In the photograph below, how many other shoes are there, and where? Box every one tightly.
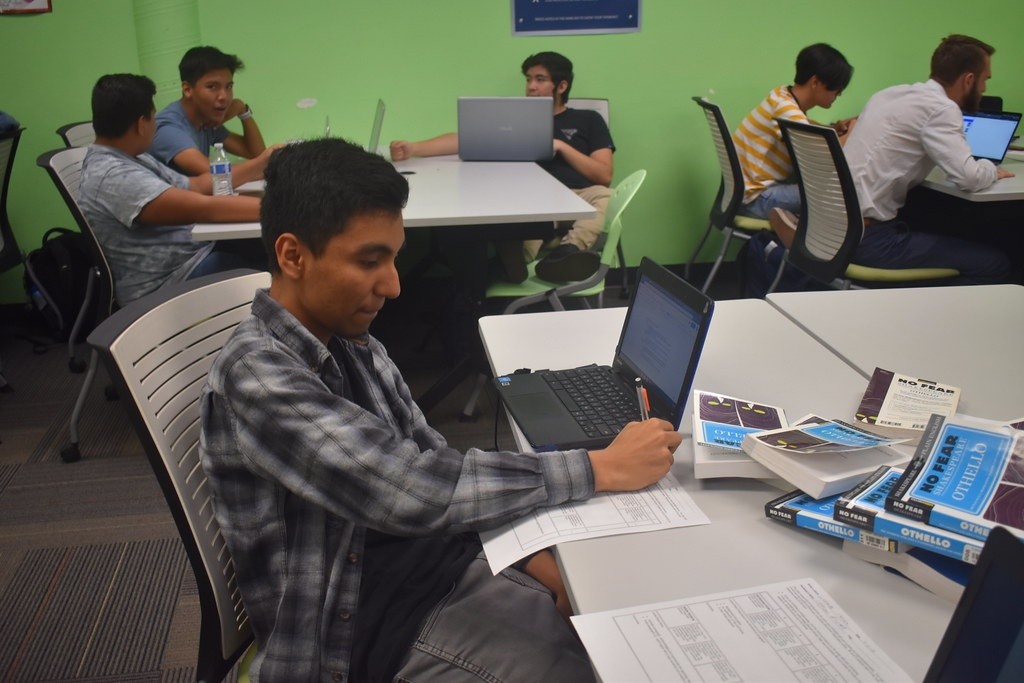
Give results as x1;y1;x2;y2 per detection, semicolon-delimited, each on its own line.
536;243;601;283
495;239;528;283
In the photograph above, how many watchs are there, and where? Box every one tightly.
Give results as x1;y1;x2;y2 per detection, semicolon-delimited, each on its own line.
238;105;253;120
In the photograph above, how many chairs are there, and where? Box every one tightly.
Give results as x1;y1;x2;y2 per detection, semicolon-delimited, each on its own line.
37;143;112;461
0;111;31;398
53;117;100;373
459;166;651;423
561;95;629;298
767;119;962;298
86;268;272;681
683;94;787;295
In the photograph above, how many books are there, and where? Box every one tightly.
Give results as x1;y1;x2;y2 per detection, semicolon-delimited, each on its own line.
691;365;1024;603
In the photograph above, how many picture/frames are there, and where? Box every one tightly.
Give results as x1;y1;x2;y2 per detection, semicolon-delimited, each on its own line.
0;0;52;15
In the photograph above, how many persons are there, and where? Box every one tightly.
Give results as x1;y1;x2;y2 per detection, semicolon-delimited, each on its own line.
389;51;616;283
76;73;287;308
145;45;267;177
196;139;684;683
824;34;1013;284
728;42;859;220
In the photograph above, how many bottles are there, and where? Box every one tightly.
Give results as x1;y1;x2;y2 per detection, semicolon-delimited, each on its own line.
209;142;233;196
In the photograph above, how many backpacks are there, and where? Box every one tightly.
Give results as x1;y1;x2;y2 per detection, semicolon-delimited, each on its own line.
23;228;100;342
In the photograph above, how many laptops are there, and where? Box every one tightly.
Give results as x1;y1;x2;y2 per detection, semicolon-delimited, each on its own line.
496;255;717;450
963;107;1024;164
457;97;557;161
368;99;385;154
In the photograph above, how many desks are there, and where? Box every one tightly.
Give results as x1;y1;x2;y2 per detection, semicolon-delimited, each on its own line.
475;297;973;682
765;281;1023;478
192;159;597;377
925;145;1024;202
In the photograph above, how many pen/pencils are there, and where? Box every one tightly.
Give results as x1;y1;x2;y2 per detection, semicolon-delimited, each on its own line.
635;377;650;421
